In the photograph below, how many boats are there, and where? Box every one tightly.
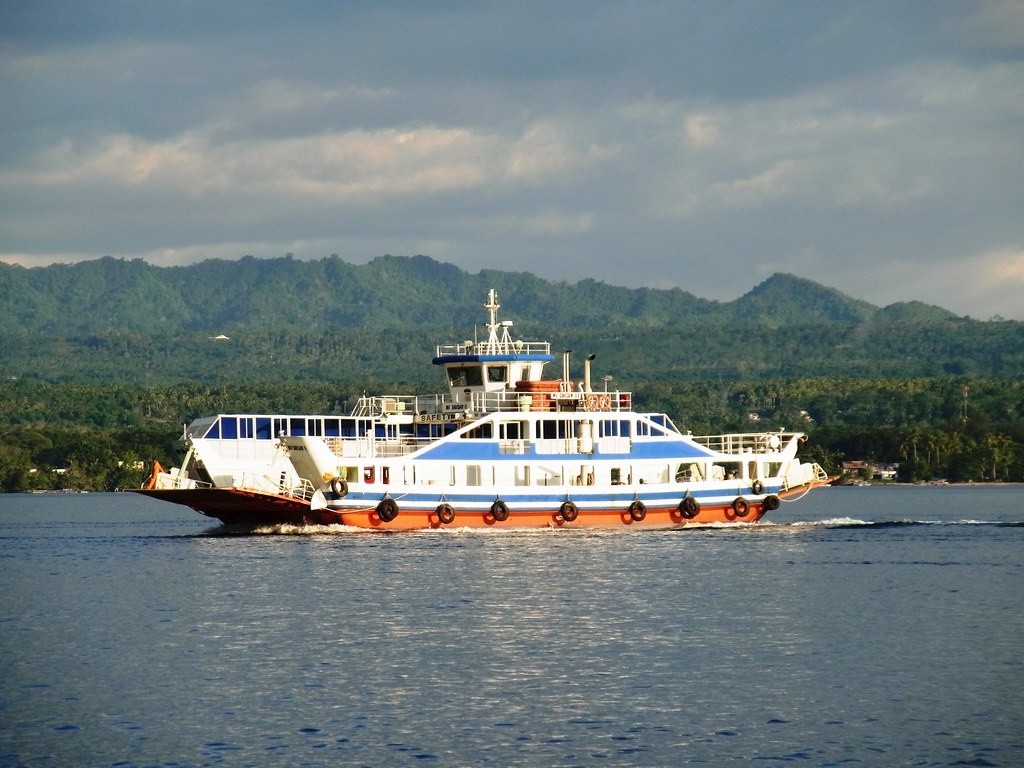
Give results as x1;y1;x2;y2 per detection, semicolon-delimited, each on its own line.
118;288;842;531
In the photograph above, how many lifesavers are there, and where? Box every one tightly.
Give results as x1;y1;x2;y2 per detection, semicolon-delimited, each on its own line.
797;435;810;452
492;501;509;521
585;393;611;412
765;495;780;510
752;481;764;495
330;476;348;497
733;497;750;517
561;502;579;522
377;499;399;522
680;497;700;519
630;501;646;521
438;504;455;524
